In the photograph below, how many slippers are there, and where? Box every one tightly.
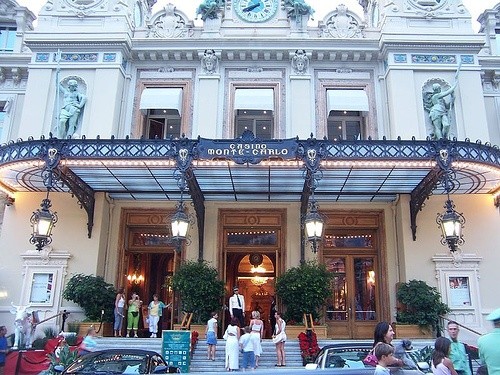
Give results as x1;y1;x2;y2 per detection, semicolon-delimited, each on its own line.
281;364;286;366
275;364;281;367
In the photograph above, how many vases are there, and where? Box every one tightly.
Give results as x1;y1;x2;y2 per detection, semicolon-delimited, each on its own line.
304;353;316;366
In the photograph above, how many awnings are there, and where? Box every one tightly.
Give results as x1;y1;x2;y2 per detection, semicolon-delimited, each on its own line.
140;88;183;118
233;88;274;116
326;89;369;120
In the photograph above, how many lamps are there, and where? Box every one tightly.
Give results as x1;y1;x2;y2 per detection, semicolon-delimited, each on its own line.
435;159;466;251
28;148;97;252
167;154;203;264
126;274;144;289
298;152;327;264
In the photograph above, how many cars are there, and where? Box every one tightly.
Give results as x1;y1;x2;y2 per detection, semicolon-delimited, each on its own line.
60;349;180;375
305;343;430;375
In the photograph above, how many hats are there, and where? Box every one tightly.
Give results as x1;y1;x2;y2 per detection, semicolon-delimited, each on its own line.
486;308;500;321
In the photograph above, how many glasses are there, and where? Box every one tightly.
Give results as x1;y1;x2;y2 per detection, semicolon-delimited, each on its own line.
235;290;238;291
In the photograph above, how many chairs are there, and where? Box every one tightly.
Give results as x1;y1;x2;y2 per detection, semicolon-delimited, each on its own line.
330;356;343;366
100;358;123;372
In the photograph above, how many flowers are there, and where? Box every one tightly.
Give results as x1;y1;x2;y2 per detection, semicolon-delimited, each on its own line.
297;330;321;356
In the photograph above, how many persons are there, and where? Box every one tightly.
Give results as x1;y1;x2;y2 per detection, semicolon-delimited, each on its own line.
125;292;143;338
272;311;287;367
57;66;87;138
204;55;217;72
146;294;172;337
79;328;98;355
430;73;459;139
238;310;264;374
432;321;472;375
205;312;218;361
294;54;306;73
224;316;240;371
113;287;127;337
477;309;500;375
373;322;403;375
229;286;246;335
0;326;9;375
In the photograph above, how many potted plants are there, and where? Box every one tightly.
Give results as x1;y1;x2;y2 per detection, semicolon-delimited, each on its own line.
390;278;447;340
60;272;119;336
167;261;227;341
274;263;336;340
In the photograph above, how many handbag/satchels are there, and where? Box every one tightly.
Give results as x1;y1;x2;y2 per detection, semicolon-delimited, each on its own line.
241;349;243;353
131;312;138;317
363;342;382;367
223;329;228;340
273;332;285;343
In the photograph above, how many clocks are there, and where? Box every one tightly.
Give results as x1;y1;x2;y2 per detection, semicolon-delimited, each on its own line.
231;0;279;23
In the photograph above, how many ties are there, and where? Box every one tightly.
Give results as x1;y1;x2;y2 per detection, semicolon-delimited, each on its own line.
237;295;241;307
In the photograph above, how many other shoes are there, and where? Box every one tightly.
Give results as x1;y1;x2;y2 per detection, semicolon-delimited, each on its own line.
134;334;138;337
154;334;157;338
127;334;129;337
150;333;154;337
